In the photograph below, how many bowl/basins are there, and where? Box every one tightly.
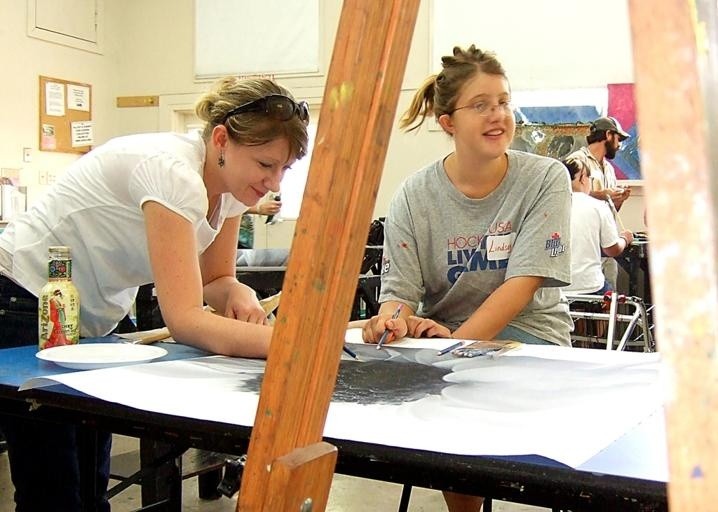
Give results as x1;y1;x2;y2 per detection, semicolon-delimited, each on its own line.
235;248;289;267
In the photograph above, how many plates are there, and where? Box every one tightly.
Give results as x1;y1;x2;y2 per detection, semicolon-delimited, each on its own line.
33;341;170;373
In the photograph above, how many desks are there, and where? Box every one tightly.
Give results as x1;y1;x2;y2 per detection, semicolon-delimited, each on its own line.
0;326;670;512
600;234;650;345
234;265;381;323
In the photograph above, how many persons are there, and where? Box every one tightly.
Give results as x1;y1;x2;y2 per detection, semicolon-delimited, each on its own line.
361;44;579;511
242;195;284;219
0;74;317;511
568;114;633;217
559;156;635;318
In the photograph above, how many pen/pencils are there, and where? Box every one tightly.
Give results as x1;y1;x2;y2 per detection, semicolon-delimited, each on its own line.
343;344;359;360
450;342;503;358
376;303;404;350
436;341;465;356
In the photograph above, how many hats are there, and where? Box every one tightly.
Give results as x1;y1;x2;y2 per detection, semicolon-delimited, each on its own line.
590;118;631;142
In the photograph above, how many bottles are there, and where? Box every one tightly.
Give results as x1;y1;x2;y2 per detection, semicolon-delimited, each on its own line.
36;244;81;347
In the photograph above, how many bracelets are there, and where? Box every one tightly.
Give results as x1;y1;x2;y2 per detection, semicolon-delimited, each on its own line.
619;235;630;247
257;203;261;214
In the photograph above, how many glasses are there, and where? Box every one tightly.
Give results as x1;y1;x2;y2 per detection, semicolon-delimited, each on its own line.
221;94;309;127
452;101;515;116
